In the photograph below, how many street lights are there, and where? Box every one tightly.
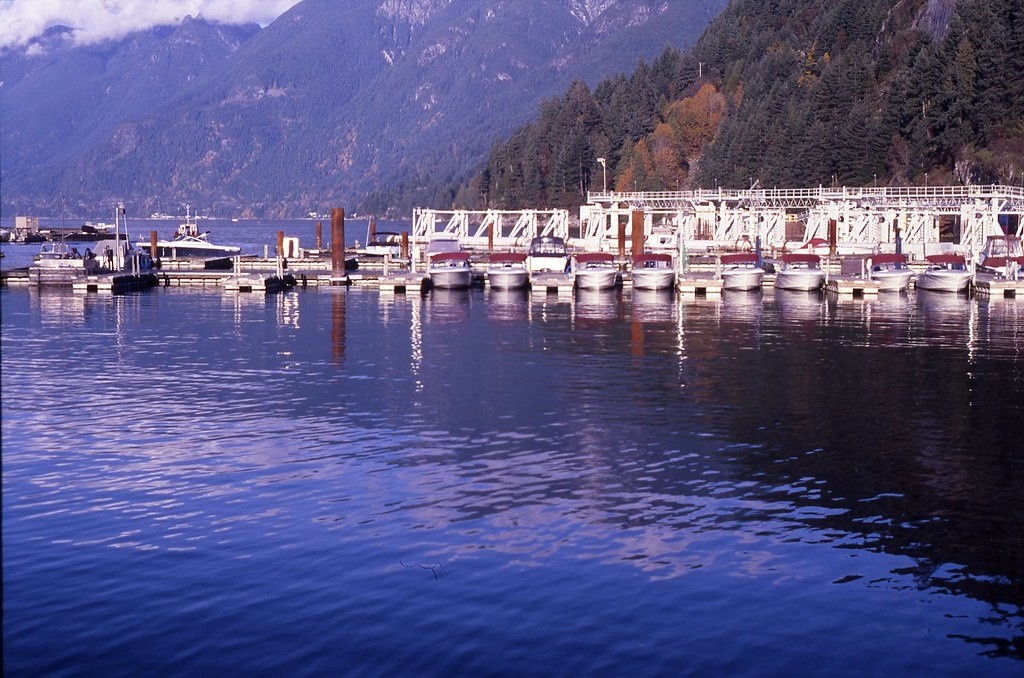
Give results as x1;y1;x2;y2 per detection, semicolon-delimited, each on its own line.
597;158;606;197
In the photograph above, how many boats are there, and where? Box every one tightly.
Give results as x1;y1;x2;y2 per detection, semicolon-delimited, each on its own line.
572;253;618;291
865;254;915;293
486;252;527;291
720;253;765;291
526;236;567;272
774;254;824;292
429;251;471;291
33;243;96;269
630;253;676;291
915;254;972;293
978;235;1024;279
136;203;241;270
365;216;403;257
